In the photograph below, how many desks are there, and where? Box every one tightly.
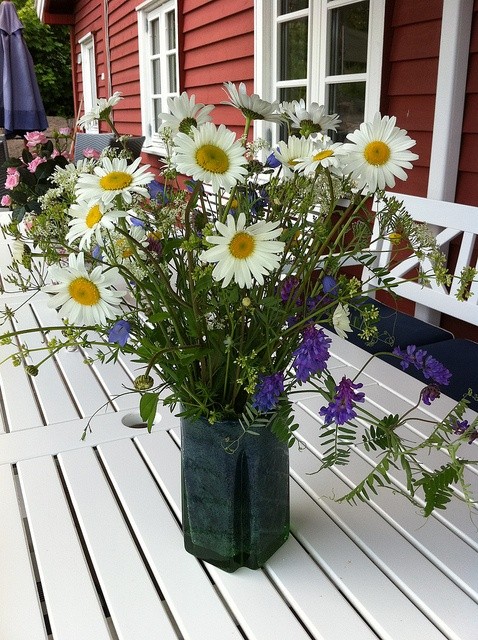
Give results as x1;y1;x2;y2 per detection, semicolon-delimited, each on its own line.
0;208;477;640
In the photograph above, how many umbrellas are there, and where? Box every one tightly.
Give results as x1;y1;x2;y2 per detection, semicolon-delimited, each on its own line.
0;2;49;138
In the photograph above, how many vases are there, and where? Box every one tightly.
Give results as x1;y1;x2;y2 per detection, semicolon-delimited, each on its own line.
14;214;47;246
181;407;291;571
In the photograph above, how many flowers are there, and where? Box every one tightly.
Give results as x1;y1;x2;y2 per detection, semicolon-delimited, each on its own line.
0;82;478;501
0;125;100;216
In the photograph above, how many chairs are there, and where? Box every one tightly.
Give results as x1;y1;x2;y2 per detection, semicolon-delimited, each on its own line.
111;136;142;168
74;132;113;168
338;295;456;363
392;338;477;413
